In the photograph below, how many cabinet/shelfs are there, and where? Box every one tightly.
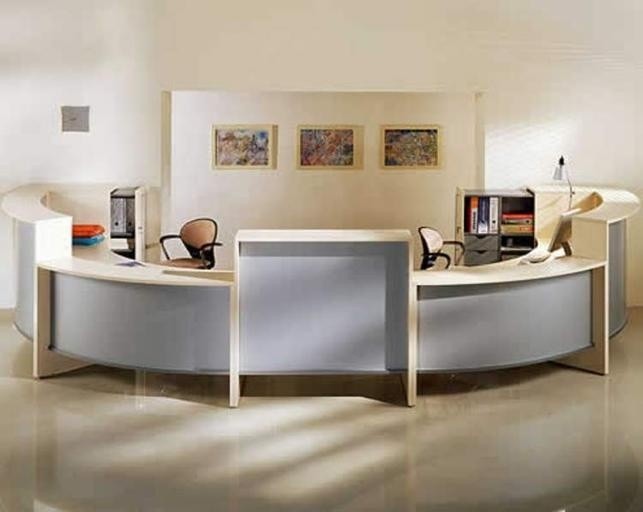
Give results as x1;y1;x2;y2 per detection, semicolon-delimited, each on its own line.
110;188;143;261
456;189;536;266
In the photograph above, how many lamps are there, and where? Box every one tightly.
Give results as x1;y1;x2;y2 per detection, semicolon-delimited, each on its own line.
554;157;577;211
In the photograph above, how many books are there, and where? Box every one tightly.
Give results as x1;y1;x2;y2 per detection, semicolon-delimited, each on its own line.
500;210;533;235
487;197;499;235
476;197;488;235
466;196;479;234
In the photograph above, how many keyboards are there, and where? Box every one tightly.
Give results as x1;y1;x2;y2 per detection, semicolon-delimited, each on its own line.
527;254;550;263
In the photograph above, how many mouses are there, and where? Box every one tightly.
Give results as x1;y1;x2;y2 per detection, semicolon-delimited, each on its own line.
520;259;529;265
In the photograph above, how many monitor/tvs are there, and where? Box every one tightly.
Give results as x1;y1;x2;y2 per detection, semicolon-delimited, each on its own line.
547;208;582;260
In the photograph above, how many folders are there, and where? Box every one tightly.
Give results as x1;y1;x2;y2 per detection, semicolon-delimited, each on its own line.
468;197;501;234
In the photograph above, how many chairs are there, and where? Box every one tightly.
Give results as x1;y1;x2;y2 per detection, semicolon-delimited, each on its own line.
417;227;466;271
161;218;223;269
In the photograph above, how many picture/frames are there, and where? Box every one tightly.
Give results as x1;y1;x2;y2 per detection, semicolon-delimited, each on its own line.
296;123;365;170
379;124;444;171
211;124;277;167
